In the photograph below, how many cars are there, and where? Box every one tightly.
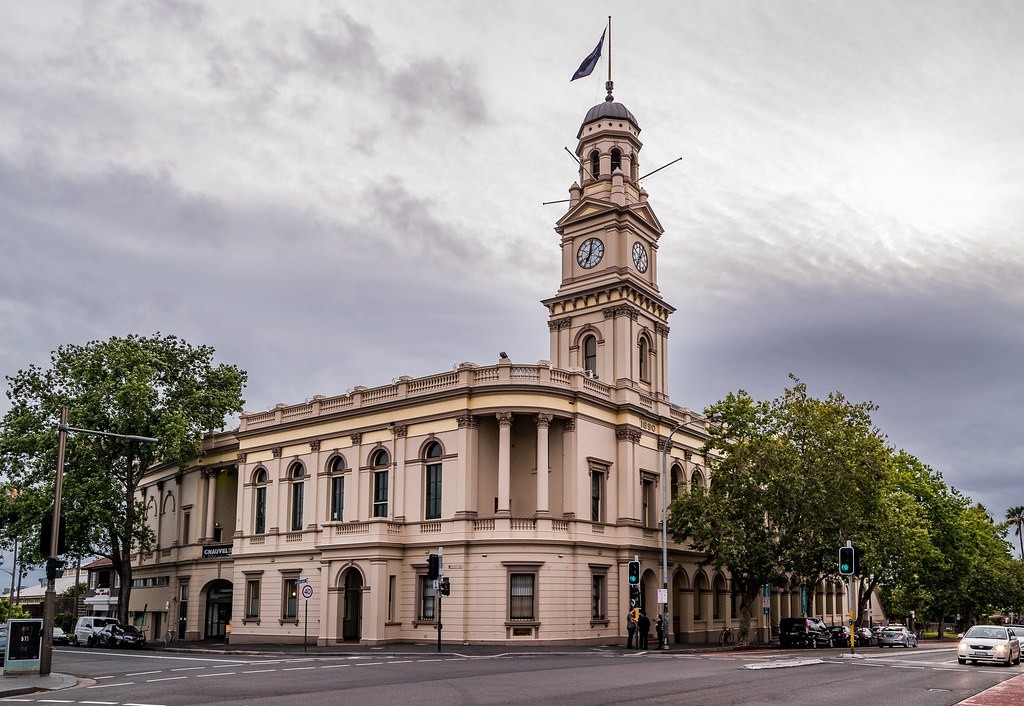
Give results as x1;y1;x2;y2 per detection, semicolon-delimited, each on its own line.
53;627;70;646
826;625;918;648
0;624;8;666
957;624;1024;667
94;624;147;649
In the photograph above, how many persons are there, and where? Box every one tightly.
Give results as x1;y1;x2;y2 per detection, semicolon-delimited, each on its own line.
654;614;663;649
627;608;636;649
637;612;650;649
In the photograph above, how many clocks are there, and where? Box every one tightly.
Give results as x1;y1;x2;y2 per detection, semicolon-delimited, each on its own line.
577;238;605;269
632;241;648;274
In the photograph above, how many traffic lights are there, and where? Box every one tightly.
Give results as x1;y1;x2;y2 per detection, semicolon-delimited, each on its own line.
630;587;638;599
839;547;854;576
426;553;439;580
441;581;451;595
629;561;640;585
55;561;66;578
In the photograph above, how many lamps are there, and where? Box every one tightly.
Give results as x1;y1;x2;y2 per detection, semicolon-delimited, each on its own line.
583;370;593;378
499;351;508;359
593;374;598;378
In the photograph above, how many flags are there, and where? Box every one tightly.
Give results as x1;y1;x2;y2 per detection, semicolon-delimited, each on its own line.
570;28;607;82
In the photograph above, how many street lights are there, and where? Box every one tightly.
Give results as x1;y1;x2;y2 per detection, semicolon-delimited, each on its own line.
662;413;722;650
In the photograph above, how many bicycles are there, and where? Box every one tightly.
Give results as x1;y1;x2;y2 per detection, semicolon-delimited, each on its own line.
719;627;734;647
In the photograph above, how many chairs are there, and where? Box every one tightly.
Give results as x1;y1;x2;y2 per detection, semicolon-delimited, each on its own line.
996;631;1005;637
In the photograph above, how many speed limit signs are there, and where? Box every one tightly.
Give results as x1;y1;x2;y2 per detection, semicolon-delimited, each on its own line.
301;585;315;599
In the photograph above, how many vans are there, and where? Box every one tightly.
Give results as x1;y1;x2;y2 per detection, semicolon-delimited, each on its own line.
779;617;834;649
73;617;122;647
